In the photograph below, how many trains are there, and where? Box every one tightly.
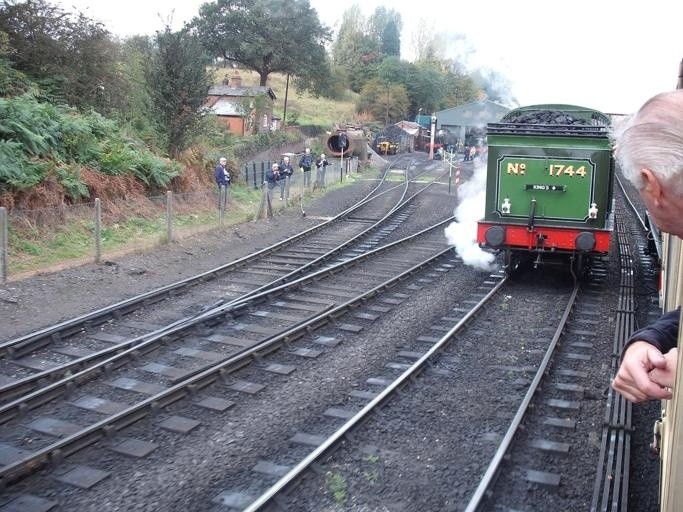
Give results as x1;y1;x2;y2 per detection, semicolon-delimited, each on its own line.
476;103;617;277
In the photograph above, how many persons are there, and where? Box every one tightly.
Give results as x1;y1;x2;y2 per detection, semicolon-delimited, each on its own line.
215;157;232;209
298;147;314;187
445;140;483;162
264;163;282;203
606;88;683;405
315;153;334;188
278;157;294;201
